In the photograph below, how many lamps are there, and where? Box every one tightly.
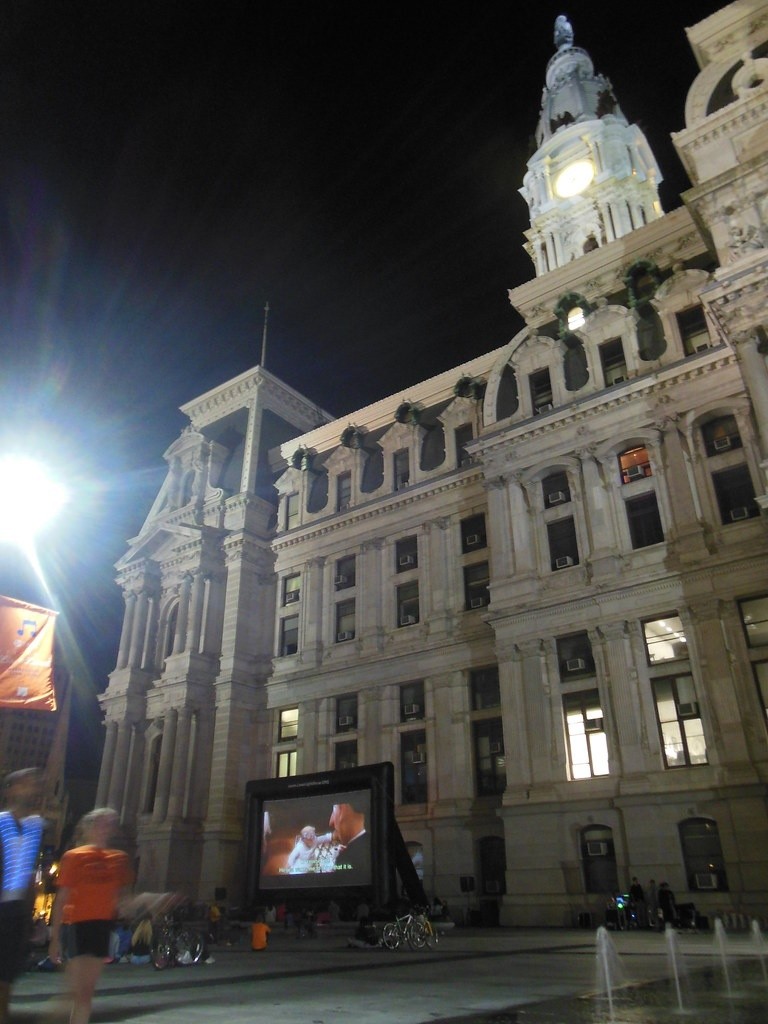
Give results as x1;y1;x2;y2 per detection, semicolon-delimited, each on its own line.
341;426;356;447
454;377;475;397
288;448;305;470
394;402;412;423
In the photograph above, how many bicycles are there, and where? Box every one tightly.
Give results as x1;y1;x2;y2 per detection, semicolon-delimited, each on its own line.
149;914;208;969
382;902;442;953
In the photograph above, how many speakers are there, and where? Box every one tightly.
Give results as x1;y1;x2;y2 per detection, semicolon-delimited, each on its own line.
460;877;474;892
215;887;227;900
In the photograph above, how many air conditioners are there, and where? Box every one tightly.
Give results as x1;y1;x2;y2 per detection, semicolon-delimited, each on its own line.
678;703;697;716
549;491;565;503
695;343;709;353
539;404;552;414
467;534;479;545
491;742;500;753
338;632;351;641
339;717;353;725
400;555;413;565
412;752;425;763
696;874;717;888
405;704;419;714
556;556;574;568
401;615;415;625
398;482;407;490
587;843;607;855
585;718;603;732
627;465;644;477
714;436;731;450
334;575;346;584
567;659;585;671
731;507;749;520
286;592;297;602
613;375;625;385
486;880;503;893
461;458;472;466
471;598;485;608
340;504;349;512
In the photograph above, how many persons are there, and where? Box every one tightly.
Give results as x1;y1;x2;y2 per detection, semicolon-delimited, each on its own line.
2;769;43;1023
284;803;365;876
50;808;134;1022
607;876;679;932
